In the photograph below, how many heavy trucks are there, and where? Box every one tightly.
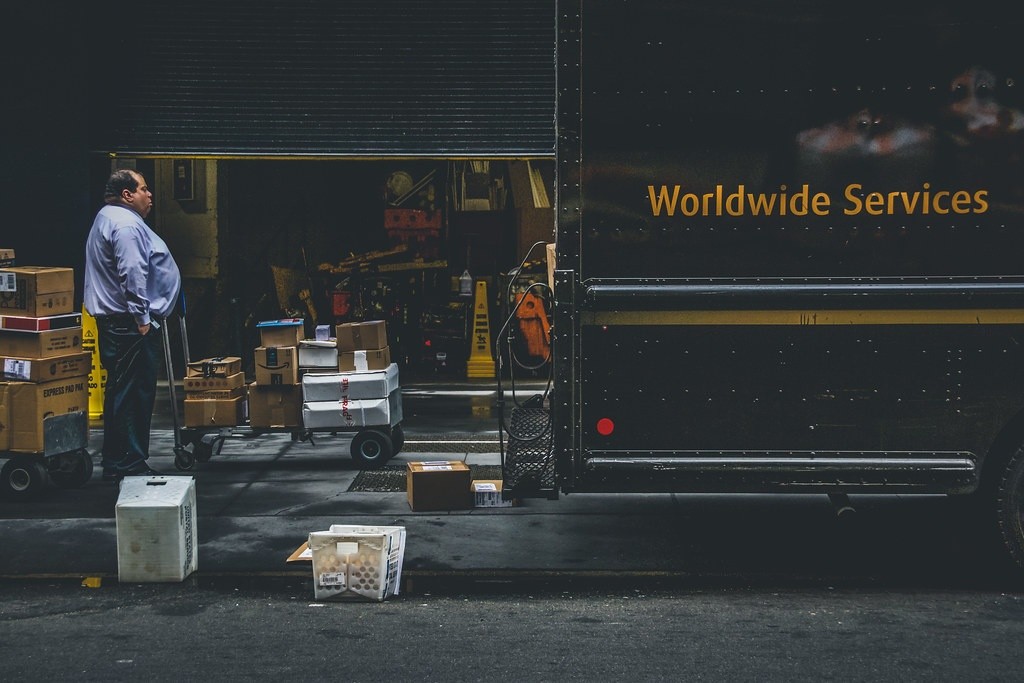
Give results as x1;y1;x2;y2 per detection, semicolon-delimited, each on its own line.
0;0;1024;590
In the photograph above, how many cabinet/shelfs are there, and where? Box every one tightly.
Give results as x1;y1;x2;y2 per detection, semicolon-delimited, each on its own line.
457;207;554;276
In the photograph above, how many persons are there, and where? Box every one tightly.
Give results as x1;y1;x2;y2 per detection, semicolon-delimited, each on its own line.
83;168;181;478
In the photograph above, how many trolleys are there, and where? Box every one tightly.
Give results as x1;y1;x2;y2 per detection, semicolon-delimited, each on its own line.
160;288;405;471
0;256;93;500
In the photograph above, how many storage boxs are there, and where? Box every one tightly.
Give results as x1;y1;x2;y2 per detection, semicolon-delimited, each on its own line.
470;477;503;507
405;459;472;513
331;290;352;317
183;320;404;429
381;207;441;244
115;476;197;583
308;524;407;602
0;249;93;454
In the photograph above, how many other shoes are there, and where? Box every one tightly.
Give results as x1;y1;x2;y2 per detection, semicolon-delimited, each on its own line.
103;468;161;481
